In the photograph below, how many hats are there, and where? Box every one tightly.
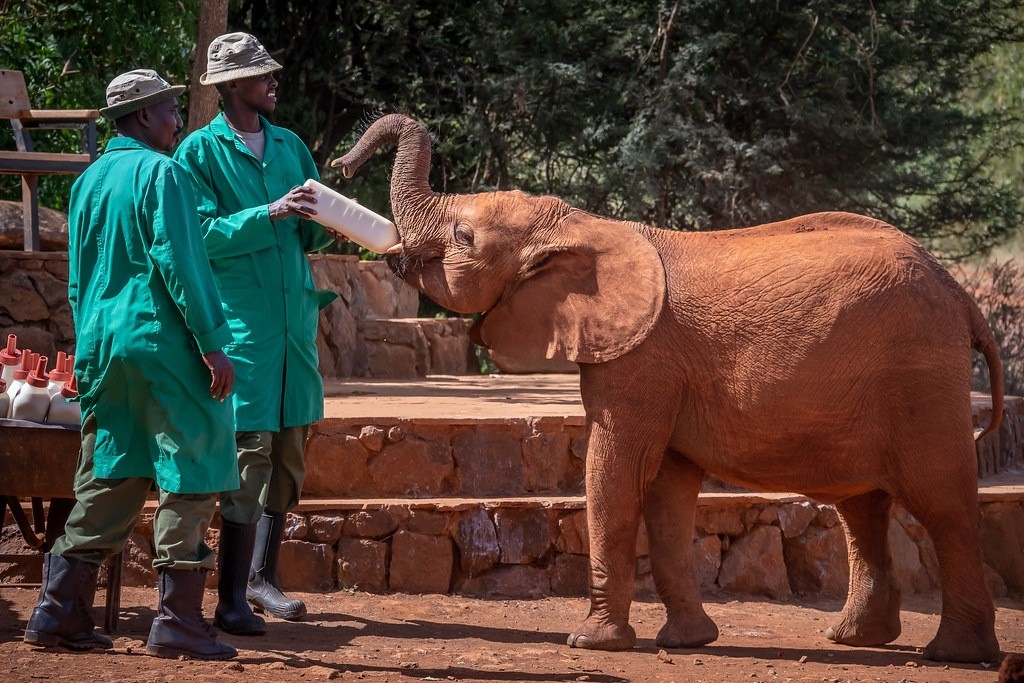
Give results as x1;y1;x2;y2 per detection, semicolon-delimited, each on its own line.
200;32;283;85
99;69;187;121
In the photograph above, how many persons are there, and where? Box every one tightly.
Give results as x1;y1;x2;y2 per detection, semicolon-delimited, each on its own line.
25;69;238;660
170;32;358;636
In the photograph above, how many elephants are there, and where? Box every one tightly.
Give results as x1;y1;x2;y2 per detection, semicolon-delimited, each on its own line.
330;113;1006;663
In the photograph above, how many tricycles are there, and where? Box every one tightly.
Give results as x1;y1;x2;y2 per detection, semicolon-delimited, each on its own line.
0;418;124;635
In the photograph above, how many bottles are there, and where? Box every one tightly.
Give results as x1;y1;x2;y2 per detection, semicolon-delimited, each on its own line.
295;179;397;255
0;334;82;425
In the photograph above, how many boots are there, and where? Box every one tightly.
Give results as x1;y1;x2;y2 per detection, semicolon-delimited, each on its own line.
245;513;307;621
145;567;238;661
213;515;267;636
24;554;114;649
